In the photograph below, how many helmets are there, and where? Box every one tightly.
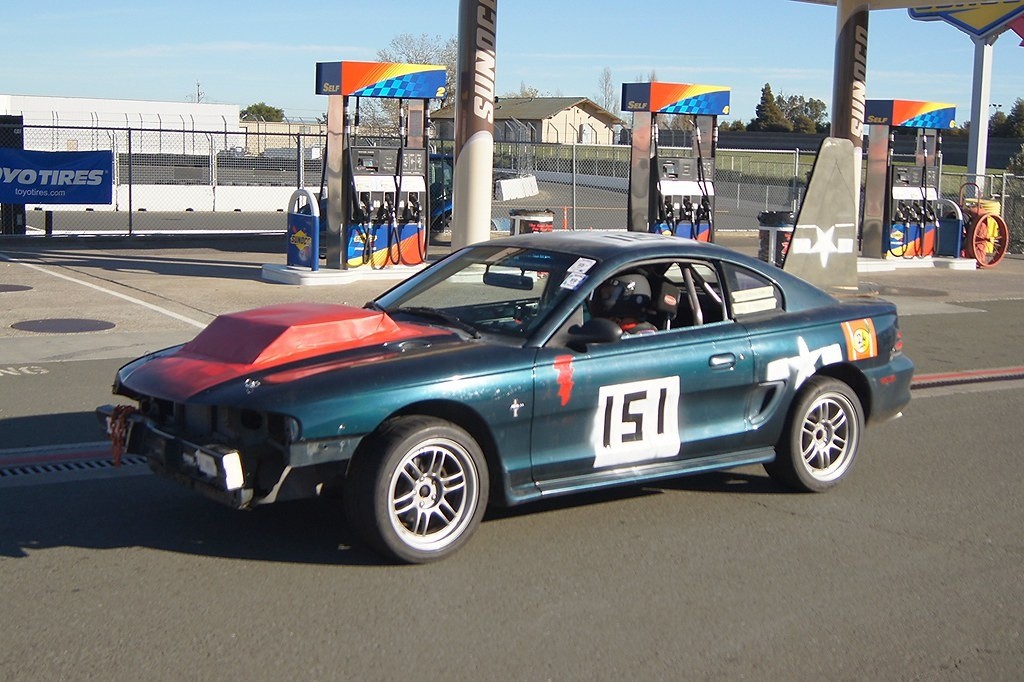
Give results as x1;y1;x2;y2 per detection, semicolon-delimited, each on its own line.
597;273;651;323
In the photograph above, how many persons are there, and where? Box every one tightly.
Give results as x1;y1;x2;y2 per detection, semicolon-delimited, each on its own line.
513;269;660;350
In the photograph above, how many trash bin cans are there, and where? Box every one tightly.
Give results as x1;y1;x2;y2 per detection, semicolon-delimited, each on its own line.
759;212;793;267
509;210;553;236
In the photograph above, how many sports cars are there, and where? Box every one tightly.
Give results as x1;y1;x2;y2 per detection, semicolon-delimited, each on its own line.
94;231;916;565
